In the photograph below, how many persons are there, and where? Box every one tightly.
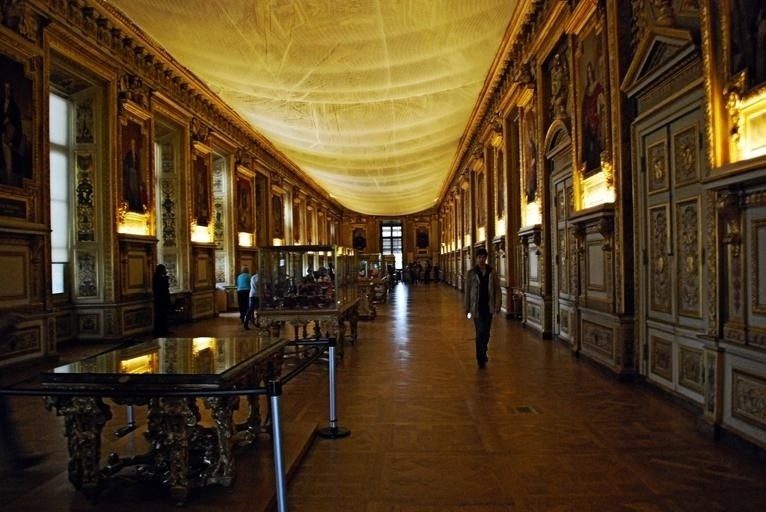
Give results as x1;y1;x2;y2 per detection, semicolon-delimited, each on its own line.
0;75;23;190
353;231;365;251
416;228;428;248
580;60;602;173
243;268;265;331
0;307;48;481
463;247;503;369
123;137;144;210
150;263;176;331
236;266;250;324
196;169;207;215
237;183;251;227
305;257;440;294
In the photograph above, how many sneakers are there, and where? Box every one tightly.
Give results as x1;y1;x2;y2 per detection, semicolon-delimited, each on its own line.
477;356;488;368
239;315;252;331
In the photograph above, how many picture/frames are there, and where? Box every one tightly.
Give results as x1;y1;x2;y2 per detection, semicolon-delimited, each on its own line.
351;226;368;253
490;133;506;236
0;25;50;236
235;164;256;247
116;98;155;235
271;183;285;247
414;222;431;249
189;140;214;244
516;83;543;227
560;0;618;210
698;0;766;184
